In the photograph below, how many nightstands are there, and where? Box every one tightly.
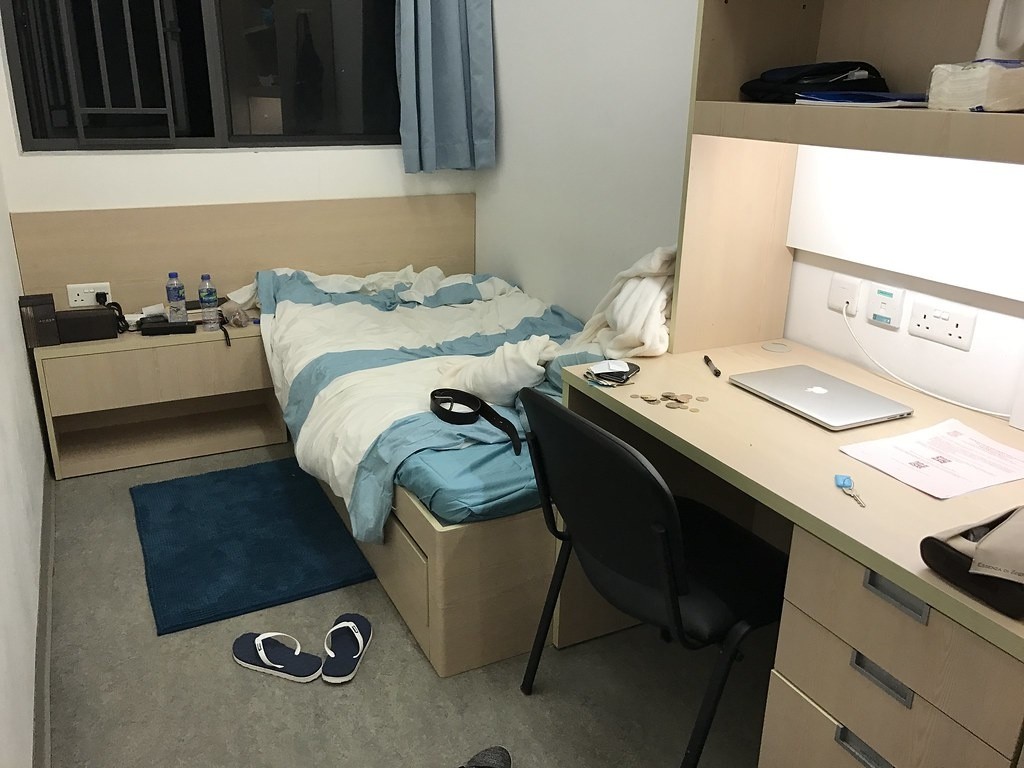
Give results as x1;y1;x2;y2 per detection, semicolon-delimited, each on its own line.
33;307;287;481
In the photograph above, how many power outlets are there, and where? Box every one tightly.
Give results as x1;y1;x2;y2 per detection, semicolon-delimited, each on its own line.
909;300;976;352
67;282;112;307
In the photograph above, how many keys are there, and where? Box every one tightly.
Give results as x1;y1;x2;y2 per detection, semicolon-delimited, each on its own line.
841;487;866;508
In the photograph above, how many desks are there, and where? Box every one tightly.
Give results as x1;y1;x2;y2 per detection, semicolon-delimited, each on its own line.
553;337;1024;663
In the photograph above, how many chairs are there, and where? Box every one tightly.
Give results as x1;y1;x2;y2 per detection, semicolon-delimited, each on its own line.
520;383;788;768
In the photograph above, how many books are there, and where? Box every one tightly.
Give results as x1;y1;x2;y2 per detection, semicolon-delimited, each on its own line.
794;91;929;108
596;362;640;383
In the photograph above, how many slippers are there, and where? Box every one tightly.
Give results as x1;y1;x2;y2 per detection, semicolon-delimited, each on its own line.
321;613;373;684
231;632;324;684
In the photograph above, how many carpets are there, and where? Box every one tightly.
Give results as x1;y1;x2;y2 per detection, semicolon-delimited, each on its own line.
129;458;377;636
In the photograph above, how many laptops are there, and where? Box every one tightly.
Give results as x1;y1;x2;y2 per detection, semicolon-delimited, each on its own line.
728;364;915;432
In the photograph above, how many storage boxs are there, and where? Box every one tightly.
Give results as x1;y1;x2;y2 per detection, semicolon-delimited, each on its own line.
55;307;118;343
18;293;60;349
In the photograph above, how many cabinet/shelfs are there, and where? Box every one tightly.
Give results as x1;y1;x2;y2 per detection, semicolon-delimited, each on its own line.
552;0;1024;768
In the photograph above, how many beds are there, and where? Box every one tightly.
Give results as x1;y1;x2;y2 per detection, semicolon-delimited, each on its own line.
255;264;607;679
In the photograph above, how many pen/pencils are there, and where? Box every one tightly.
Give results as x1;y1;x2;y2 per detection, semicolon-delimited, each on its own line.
704;355;721;377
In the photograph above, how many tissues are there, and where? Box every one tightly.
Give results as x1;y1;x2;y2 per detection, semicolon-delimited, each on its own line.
926;57;1024;113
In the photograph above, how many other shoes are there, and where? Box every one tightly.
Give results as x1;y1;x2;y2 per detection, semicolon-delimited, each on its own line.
456;746;512;768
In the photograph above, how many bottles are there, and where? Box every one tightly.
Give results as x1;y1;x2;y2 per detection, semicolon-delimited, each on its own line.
198;274;220;332
165;272;188;322
